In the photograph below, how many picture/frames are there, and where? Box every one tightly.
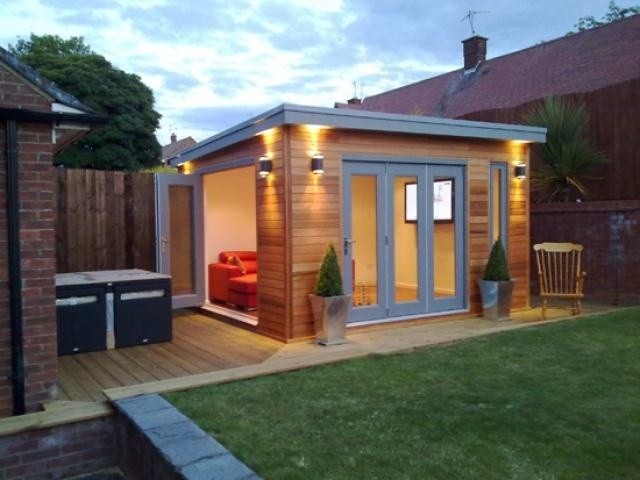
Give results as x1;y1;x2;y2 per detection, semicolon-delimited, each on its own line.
404;178;455;223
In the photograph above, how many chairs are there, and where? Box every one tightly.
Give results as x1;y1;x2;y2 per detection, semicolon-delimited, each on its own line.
533;242;587;321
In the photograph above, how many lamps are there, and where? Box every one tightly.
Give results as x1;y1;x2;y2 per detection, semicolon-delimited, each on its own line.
177;164;185;175
311;156;325;174
256;157;273;177
515;164;527;180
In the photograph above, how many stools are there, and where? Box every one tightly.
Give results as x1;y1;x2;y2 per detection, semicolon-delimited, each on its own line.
228;273;259;310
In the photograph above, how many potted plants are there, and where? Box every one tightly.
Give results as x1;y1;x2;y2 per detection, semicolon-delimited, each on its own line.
477;236;516;322
307;240;353;345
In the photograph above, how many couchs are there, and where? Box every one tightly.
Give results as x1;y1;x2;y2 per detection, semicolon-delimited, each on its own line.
208;251;259;308
